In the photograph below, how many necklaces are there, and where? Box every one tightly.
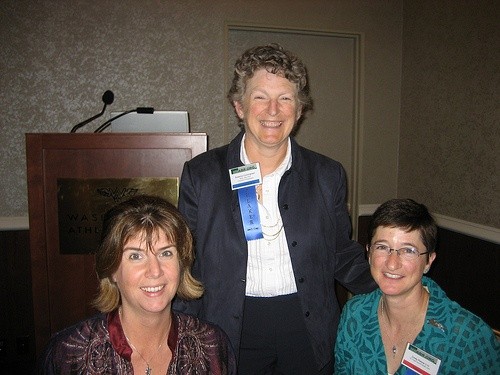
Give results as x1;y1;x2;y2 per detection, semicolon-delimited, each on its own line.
256;189;263;202
381;297;428;357
258;217;284;240
117;310;172;375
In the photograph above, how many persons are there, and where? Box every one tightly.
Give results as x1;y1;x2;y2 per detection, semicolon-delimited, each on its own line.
38;194;237;375
333;198;500;375
171;41;381;375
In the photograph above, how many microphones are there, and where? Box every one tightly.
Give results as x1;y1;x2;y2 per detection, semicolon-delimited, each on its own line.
71;90;114;132
93;107;155;133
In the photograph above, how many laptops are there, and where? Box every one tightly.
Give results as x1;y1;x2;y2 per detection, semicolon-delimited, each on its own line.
110;110;189;133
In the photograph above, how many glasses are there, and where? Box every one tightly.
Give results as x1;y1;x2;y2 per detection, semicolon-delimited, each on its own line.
368;243;430;261
